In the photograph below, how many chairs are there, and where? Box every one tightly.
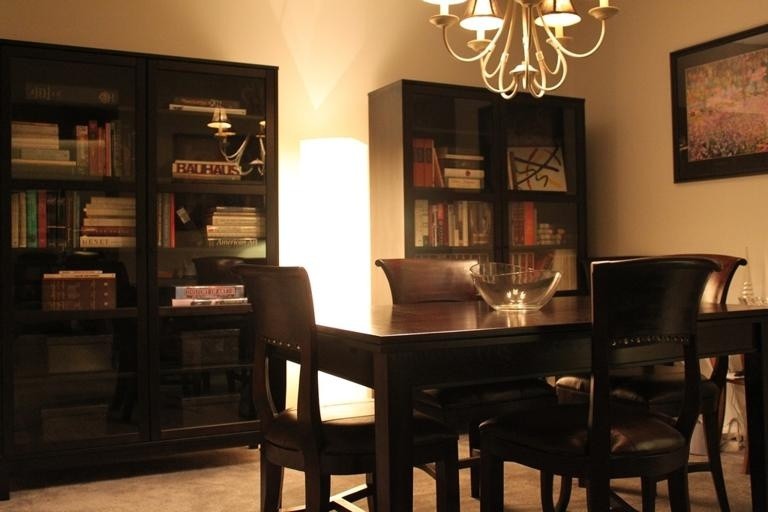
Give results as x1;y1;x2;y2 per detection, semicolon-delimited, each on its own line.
474;252;725;512
235;261;460;512
376;256;560;512
554;253;748;512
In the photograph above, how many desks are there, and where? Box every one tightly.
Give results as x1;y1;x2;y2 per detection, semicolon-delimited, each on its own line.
267;296;768;511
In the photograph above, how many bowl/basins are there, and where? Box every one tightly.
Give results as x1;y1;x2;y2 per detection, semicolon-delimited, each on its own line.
469;259;564;316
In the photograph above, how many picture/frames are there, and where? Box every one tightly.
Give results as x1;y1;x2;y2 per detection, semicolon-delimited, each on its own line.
668;23;767;187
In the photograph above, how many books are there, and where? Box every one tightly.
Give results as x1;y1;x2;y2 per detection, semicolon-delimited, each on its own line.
10;189;266;261
44;267;254;310
410;128;576;294
13;114;131;178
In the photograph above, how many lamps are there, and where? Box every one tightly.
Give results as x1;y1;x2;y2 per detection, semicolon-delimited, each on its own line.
420;0;620;102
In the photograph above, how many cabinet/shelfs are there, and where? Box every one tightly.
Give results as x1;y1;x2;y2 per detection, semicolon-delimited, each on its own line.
368;79;586;306
1;38;280;503
